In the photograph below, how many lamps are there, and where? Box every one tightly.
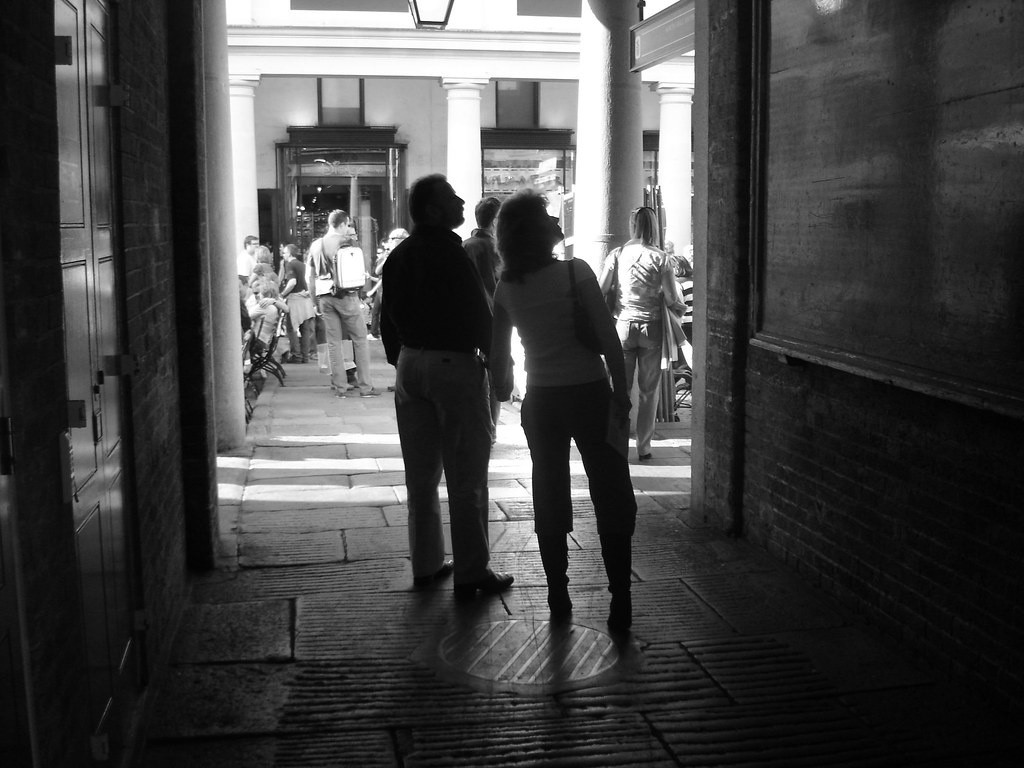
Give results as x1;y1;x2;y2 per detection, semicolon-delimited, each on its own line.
409;0;455;31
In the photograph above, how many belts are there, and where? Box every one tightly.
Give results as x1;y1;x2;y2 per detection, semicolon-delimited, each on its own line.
404;344;474;352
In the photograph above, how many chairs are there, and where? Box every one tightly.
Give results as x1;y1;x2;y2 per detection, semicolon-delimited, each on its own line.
243;313;288;422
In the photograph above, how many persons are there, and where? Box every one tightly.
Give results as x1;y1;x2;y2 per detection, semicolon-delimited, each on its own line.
464;198;504;452
238;209;408;398
381;174;514;604
662;240;693;358
488;189;636;642
598;206;676;459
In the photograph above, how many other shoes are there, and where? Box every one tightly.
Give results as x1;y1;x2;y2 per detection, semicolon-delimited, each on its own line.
454;573;514;599
331;382;354;390
388;385;396;390
286;356;308;364
360;387;383;397
334;390;346;398
414;562;454;586
608;590;633;629
548;576;572;612
639;452;653;460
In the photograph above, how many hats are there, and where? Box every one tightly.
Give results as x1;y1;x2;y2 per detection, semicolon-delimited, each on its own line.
389;228;410;238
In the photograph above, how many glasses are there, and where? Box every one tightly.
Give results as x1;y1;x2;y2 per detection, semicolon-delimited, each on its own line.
634;206;655;224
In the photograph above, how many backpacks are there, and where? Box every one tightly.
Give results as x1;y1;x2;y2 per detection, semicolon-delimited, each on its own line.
334;241;365;291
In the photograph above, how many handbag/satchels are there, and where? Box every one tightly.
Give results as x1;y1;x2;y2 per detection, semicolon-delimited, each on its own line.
602;250;618;312
569;259;602;354
